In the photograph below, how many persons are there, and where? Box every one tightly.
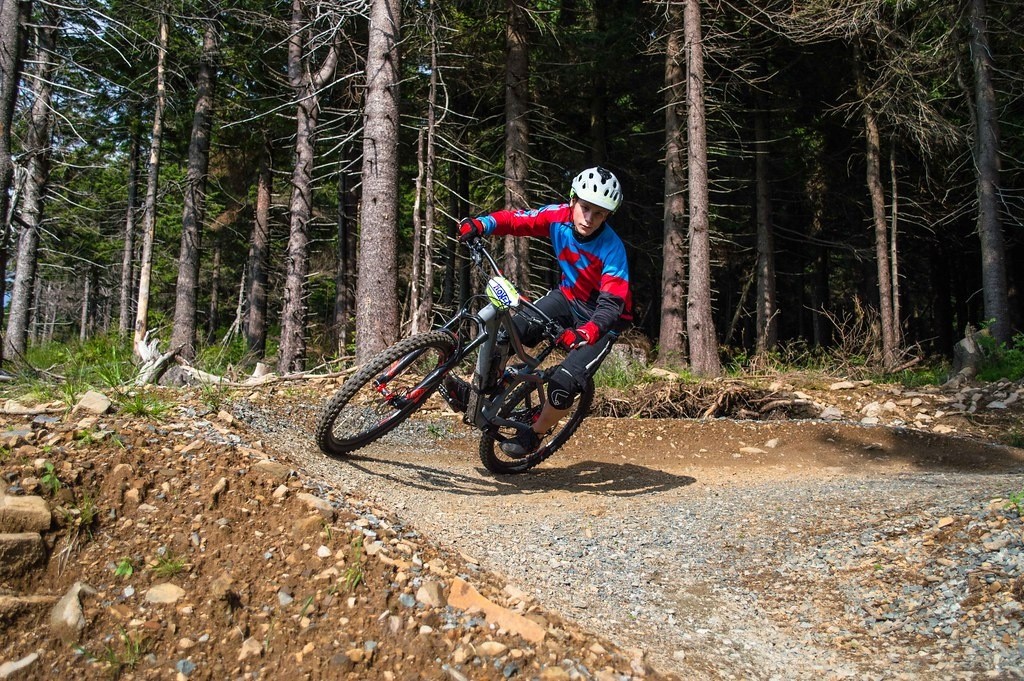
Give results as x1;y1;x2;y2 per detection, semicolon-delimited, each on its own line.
440;165;634;460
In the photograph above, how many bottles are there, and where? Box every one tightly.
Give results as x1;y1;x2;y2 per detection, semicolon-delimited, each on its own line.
488;366;517;400
497;330;509;379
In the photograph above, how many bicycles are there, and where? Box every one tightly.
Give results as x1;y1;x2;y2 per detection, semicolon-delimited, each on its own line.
314;235;597;475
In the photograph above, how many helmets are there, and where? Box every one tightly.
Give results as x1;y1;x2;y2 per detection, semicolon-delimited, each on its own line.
569;165;623;215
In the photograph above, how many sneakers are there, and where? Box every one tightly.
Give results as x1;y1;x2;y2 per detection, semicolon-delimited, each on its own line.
443;373;471;413
499;427;544;459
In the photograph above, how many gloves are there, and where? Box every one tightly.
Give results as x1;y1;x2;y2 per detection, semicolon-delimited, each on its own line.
455;217;484;243
554;327;588;353
